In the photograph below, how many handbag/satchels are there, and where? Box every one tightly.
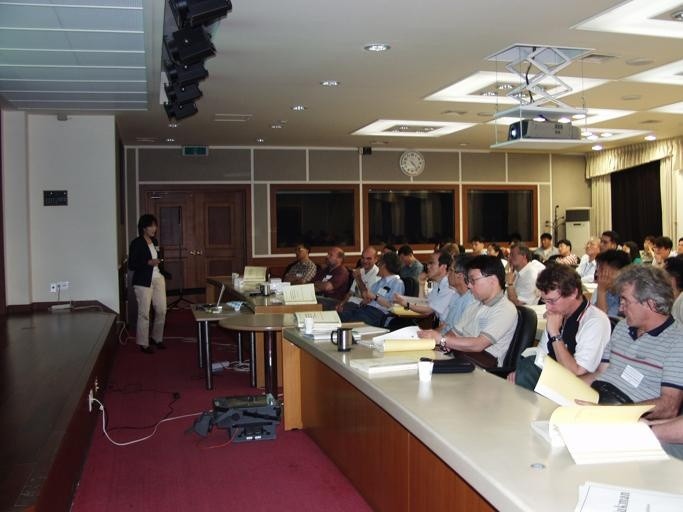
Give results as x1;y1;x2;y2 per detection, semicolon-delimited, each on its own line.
421;358;474;373
453;347;498;369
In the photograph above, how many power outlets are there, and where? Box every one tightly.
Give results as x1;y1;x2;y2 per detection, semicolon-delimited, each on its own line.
88;389;94;413
50;280;69;292
95;376;99;392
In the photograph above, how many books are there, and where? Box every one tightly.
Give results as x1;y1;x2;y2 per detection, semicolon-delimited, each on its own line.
349;351;422;373
360;325;436;351
302;332;361;342
526;402;675;466
519;348;600;412
388;294;430;317
241;265;268;282
275;283;316;306
292;310;341;333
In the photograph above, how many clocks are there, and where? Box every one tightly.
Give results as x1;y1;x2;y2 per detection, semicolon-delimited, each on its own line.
399;151;425;176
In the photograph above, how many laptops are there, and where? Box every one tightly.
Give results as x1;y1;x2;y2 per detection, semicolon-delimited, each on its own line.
196;284;227;312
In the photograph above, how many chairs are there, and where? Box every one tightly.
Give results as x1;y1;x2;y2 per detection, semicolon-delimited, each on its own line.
487;306;536;379
283;262;321;284
316;267;354;311
383;276;420;330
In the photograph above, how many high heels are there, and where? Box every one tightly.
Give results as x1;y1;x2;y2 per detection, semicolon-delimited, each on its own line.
139;343;153;353
150;337;165;348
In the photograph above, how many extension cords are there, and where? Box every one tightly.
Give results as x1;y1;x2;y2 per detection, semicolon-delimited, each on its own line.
52;306;72;311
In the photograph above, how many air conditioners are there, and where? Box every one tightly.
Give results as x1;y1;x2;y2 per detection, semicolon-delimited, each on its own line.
565;207;591;259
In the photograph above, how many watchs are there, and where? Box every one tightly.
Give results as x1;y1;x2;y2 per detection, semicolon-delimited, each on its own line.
438;334;449;348
402;301;411;311
374;293;381;303
547;335;564;343
505;282;513;289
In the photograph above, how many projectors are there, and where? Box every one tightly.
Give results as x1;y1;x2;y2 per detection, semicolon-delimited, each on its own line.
508;120;581;141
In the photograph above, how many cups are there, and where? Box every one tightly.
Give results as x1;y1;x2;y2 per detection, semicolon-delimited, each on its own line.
230;273;238;285
329;328;352;352
417;361;433;383
303;317;312;336
259;284;270;298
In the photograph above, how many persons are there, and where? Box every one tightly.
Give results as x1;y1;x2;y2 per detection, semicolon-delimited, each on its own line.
670;237;683;259
437;252;474;352
335;246;382;322
621;241;644;264
508;263;613;399
535;231;558;264
600;230;621;254
286;242;318;289
574;237;599;284
641;235;659;265
393;249;454;346
652;235;677;269
341;251;405;332
579;262;683;423
309;246;350;313
469;234;489;257
486;243;515;270
442;243;465;261
384;245;424;292
594;250;636;316
418;254;519;372
553;240;580;270
502;241;549;306
128;213;168;355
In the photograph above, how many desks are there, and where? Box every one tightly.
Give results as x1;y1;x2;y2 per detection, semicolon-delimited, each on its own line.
191;301;256;390
220;312;297;398
206;276;323;388
282;321;683;512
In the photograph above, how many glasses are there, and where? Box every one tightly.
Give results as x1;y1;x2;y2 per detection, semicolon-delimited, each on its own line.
541;292;565;304
295;248;307;251
467;272;494;284
